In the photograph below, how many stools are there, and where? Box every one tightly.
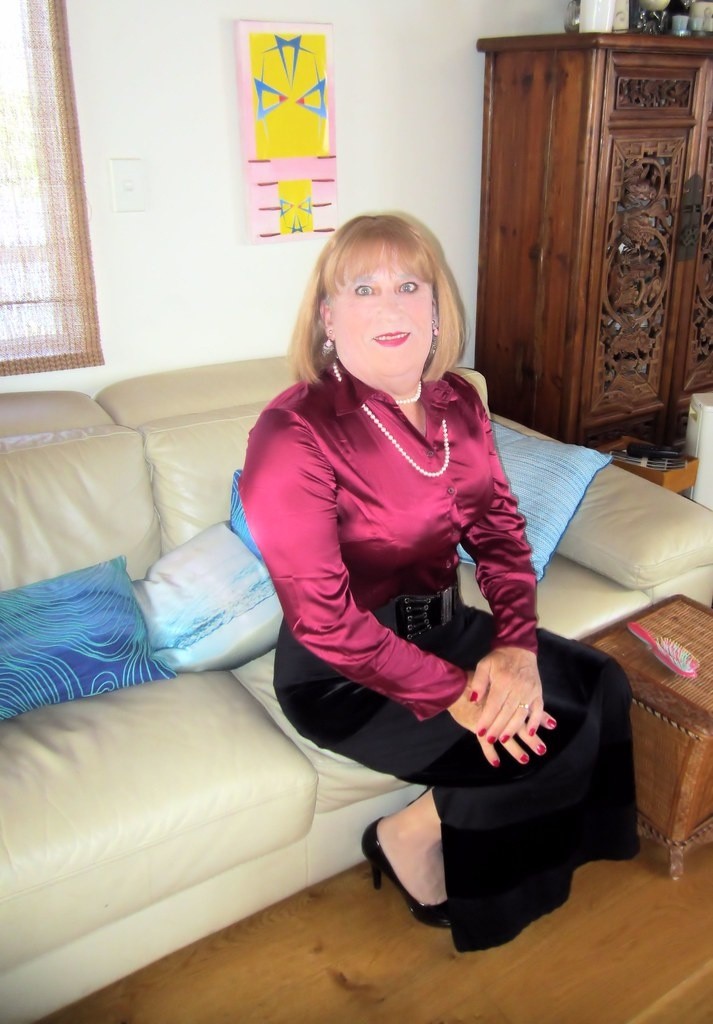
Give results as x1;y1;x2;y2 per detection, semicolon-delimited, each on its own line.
567;594;712;884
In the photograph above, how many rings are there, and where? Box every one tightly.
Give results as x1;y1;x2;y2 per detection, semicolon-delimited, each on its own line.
519;704;533;709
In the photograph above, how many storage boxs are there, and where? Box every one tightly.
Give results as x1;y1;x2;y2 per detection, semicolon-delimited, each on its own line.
595;434;698;494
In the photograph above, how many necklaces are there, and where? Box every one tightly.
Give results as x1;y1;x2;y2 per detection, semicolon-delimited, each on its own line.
333;361;449;476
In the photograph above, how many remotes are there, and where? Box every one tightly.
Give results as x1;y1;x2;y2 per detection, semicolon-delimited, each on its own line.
627;442;679;460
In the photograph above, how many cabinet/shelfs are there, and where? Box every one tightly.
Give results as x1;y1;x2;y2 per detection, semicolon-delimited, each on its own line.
473;34;713;456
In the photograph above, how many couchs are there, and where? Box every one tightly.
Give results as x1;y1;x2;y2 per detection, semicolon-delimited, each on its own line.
1;380;713;1024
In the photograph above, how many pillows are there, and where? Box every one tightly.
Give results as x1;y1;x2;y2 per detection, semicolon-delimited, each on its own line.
460;418;611;583
1;555;182;715
134;467;287;675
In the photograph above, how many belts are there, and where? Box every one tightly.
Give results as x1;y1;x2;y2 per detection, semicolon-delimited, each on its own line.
373;584;463;640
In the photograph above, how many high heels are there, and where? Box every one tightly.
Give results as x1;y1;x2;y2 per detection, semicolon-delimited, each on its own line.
362;817;451;926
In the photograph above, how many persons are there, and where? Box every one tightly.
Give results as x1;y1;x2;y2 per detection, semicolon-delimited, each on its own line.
237;213;645;951
